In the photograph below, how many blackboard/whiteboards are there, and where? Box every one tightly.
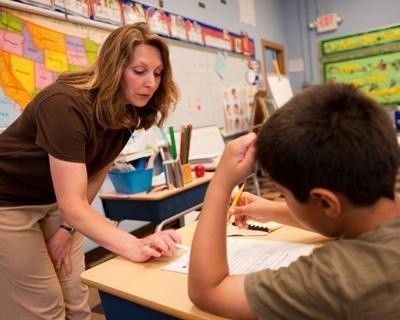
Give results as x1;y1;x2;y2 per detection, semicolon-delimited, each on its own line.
157;35;247;136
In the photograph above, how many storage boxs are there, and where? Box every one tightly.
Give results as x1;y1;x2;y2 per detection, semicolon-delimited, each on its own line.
108;168;155;194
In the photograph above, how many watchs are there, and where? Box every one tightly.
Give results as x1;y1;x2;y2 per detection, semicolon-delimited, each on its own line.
60;225;76;234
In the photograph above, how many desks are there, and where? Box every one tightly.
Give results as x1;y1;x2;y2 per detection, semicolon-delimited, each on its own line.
99;157;261;233
81;206;336;320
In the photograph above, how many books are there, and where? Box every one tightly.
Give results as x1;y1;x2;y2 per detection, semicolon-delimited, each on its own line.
231;213;284;232
167;124;225;171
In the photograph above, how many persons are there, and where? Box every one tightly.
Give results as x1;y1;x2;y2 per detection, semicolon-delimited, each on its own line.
0;19;184;320
187;78;400;320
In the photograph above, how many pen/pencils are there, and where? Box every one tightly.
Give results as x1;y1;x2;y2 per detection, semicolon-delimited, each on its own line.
175;243;188;250
226;184;245;222
167;124;192;165
